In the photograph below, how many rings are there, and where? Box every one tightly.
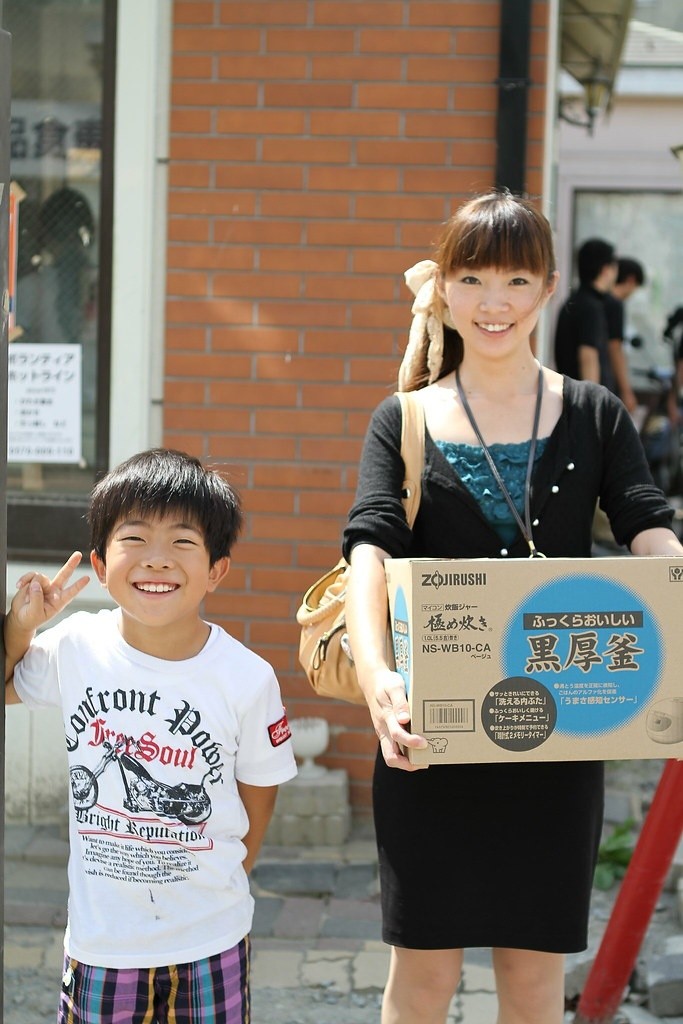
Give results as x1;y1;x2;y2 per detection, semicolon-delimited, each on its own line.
378;733;386;741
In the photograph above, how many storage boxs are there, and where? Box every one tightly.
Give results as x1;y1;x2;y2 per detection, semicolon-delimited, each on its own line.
384;556;683;764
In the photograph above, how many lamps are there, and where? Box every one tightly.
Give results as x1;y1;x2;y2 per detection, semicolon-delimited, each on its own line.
557;57;611;138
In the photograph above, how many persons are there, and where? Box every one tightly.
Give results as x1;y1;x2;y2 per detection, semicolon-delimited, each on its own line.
5;444;303;1024
9;240;69;344
661;304;683;425
338;186;683;1024
553;238;618;396
31;178;92;268
605;256;644;414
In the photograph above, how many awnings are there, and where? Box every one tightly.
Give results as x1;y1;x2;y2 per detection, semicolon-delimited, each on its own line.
556;1;633;119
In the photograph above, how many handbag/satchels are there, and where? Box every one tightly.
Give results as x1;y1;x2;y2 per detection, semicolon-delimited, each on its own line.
295;390;426;706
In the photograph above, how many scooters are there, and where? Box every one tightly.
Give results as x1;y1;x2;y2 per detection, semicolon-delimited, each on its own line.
639;367;682;496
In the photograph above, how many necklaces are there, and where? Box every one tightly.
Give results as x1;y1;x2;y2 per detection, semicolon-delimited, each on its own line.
455;357;546;557
